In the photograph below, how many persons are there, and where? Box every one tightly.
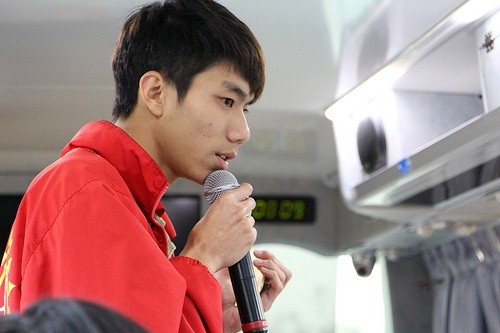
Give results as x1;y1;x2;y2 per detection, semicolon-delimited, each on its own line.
0;297;149;333
0;0;291;333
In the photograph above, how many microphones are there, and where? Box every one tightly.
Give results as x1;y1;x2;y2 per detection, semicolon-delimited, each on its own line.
203;169;270;333
